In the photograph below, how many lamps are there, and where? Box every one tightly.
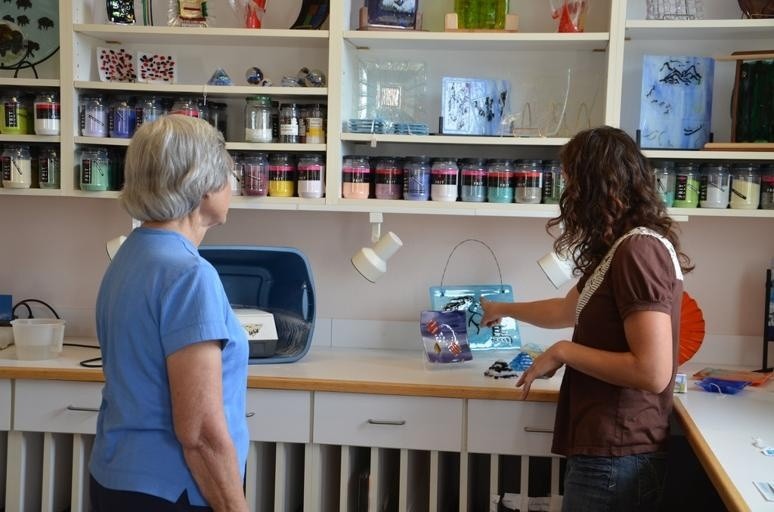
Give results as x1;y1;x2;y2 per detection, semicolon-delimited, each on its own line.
536;217;593;289
105;217;140;262
349;213;404;285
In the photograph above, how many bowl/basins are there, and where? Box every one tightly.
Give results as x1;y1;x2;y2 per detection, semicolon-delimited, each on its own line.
9;317;67;358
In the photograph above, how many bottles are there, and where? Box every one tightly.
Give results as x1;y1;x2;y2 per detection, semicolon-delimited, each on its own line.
431;156;459;202
699;161;728;207
545;160;565;204
676;160;699;208
729;164;761;209
488;158;514;204
374;156;401;199
76;94;326;198
650;159;676;207
458;157;487;202
514;159;544;204
761;165;773;209
343;155;370;200
402;154;431;201
2;87;60;190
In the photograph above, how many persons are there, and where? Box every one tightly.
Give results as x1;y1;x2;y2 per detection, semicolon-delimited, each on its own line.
479;126;695;512
87;114;250;512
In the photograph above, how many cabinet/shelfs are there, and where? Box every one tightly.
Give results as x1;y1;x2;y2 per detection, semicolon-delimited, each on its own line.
618;1;773;218
338;0;619;219
0;0;72;195
72;0;338;210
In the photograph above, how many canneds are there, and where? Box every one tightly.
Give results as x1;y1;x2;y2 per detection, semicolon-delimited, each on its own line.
0;87;61;188
342;154;566;205
649;158;774;210
78;92;327;199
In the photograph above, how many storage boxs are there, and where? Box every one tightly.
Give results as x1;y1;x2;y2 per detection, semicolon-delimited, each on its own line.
196;244;316;365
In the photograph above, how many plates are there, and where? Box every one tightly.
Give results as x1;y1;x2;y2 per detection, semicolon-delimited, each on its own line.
95;46;179;85
418;309;474;364
691;367;772;395
0;1;57;69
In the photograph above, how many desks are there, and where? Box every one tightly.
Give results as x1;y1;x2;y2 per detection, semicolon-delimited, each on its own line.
0;328;772;511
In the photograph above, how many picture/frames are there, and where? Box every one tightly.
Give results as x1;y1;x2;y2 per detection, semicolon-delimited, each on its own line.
452;0;510;33
357;0;424;32
